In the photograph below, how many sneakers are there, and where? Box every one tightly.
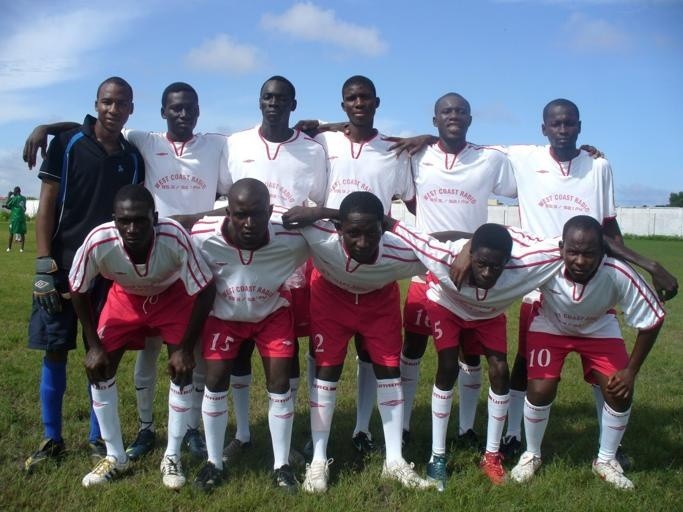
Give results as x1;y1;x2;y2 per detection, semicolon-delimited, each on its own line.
591;445;636;493
287;429;525;467
301;458;335;496
81;454;135;487
272;464;301;494
159;453;186;491
24;439;70;475
510;451;544;486
379;459;433;491
80;429;252;469
426;453;449;493
7;248;23;253
192;461;228;496
480;449;507;485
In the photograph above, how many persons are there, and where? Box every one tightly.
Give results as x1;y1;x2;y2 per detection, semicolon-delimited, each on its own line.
217;78;328;465
2;187;26;252
164;190;479;494
291;92;606;451
381;97;635;470
303;76;416;458
22;76;146;473
451;215;666;491
281;203;679;488
185;177;313;492
21;81;351;461
68;182;217;491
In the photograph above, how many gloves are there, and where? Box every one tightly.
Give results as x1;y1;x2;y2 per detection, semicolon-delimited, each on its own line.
32;255;72;317
